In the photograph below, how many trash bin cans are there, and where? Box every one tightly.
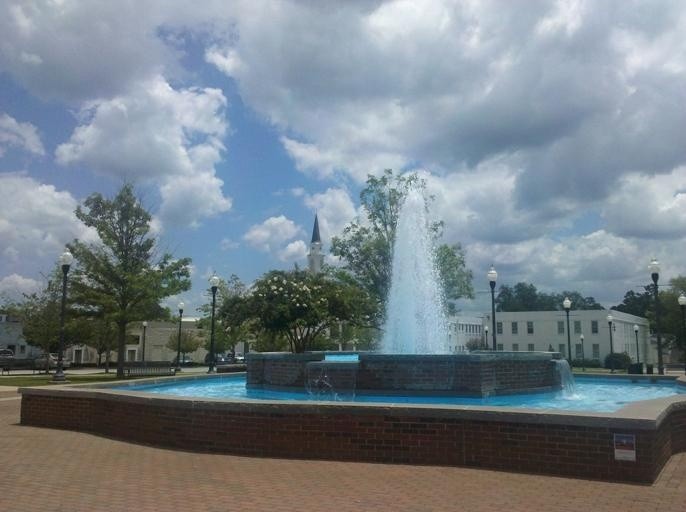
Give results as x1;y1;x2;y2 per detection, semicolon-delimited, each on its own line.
647;363;653;374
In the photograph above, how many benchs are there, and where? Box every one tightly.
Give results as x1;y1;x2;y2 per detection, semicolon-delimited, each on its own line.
3;358;41;375
124;359;176;378
666;364;686;374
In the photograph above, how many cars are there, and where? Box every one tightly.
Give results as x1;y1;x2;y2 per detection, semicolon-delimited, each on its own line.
32;352;71;371
205;351;249;365
172;355;194;367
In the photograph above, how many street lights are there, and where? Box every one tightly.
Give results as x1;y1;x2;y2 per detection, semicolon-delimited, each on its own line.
207;276;220;375
650;257;664;375
562;296;572;362
174;302;184;371
633;323;640;364
579;333;586;371
485;262;498;352
53;248;75;381
141;320;146;362
606;313;615;373
676;294;685;343
